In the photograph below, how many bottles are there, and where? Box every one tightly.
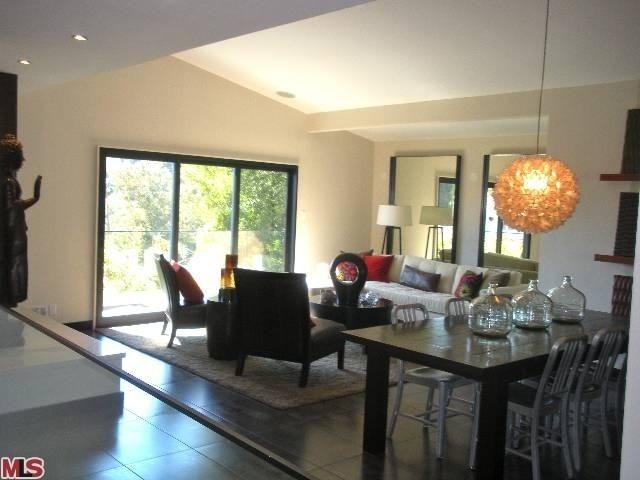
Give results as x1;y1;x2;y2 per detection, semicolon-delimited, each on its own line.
467;275;587;336
357;290;380;305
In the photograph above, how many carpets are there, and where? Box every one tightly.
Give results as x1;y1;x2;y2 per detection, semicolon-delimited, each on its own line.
96;321;410;410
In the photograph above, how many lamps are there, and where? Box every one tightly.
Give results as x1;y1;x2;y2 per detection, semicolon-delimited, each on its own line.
376;205;413;255
491;1;580;234
420;206;452;262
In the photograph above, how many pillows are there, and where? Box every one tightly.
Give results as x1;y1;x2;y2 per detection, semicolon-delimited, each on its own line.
398;264;440;292
454;270;482;299
340;249;373;260
364;255;395;283
488;271;512;287
170;260;204;303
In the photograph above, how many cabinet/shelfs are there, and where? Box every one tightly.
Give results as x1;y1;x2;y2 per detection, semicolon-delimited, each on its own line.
593;173;639;265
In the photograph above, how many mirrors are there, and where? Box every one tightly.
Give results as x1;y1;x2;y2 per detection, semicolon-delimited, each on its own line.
477;154;540;268
386;155;461;264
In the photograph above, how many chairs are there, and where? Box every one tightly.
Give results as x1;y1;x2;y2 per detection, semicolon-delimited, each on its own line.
525;327;626;472
233;267;346;387
445;297;476;420
469;333;588;480
391;303;479;459
584;350;629;455
154;253;207;348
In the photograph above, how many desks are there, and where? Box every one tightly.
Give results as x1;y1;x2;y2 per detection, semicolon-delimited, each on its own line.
207;296;238;360
338;308;630;480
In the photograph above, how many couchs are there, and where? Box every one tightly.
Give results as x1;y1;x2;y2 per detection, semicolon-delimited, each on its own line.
440;250;538;284
360;254;538;315
